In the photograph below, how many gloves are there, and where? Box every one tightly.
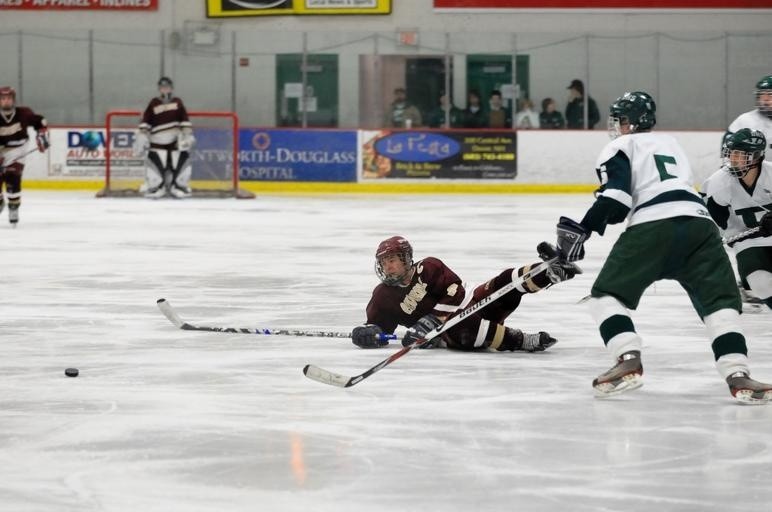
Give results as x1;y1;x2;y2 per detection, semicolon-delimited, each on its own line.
401;312;445;349
131;134;149;156
36;127;50;153
555;216;587;263
352;323;389;349
758;212;772;238
178;135;197;154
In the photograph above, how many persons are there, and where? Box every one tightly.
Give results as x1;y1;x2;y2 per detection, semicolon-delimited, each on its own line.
137;77;195;198
697;128;772;309
0;86;50;222
352;236;575;354
386;80;600;130
557;91;772;403
721;74;772;305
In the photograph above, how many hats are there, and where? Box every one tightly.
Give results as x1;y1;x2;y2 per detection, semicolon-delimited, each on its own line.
566;79;583;90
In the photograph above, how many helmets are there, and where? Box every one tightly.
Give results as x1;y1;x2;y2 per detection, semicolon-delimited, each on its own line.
0;86;16;100
158;77;174;86
608;90;657;119
755;75;772;91
720;128;767;152
374;236;413;261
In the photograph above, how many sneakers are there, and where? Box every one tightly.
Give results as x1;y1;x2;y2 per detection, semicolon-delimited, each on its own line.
592;350;644;387
537;241;576;286
725;370;772;399
736;279;765;306
9;208;18;224
520;330;549;354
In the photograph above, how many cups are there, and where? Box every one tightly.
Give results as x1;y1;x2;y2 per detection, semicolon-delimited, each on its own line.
406;119;412;128
